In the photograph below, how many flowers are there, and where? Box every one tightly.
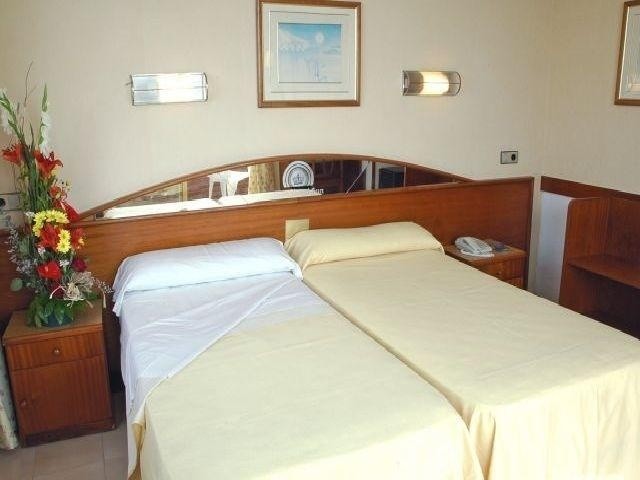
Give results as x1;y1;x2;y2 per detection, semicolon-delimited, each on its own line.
0;58;105;327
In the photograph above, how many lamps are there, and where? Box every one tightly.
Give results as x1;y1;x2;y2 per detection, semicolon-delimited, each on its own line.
129;71;209;106
401;68;461;97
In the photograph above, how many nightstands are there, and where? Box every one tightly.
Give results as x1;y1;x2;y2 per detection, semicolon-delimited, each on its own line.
4;299;119;449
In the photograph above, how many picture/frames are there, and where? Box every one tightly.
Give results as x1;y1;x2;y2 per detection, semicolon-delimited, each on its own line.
612;0;640;106
255;0;361;107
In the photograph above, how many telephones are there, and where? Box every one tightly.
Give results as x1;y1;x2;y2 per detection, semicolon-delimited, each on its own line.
455;237;492;254
